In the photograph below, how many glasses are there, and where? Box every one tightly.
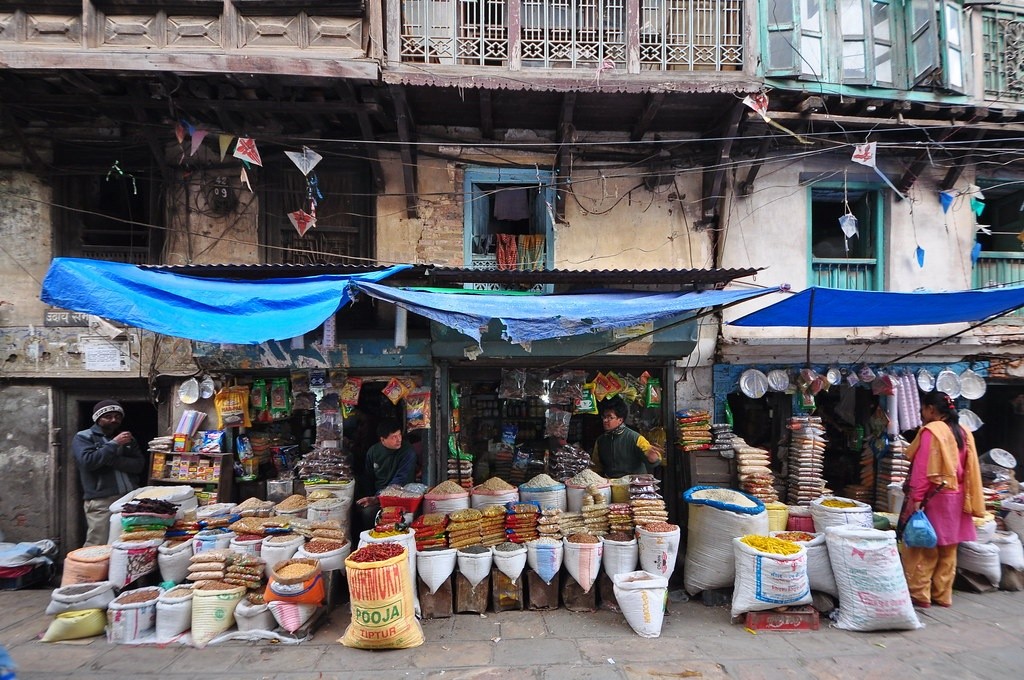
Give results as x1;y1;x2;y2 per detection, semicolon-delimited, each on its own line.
603;415;620;421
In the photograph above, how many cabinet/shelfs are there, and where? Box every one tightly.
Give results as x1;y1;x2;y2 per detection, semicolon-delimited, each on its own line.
678;448;738;494
233;414;299;501
148;450;234;505
467;394;584;454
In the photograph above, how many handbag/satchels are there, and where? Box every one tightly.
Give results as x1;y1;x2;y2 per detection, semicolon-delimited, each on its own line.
902;509;937;548
895;506;912;544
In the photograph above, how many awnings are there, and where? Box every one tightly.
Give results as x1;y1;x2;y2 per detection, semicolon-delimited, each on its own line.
728;285;1024;368
39;257;790;367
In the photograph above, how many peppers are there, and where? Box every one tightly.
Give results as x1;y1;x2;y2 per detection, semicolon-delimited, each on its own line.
347;542;405;562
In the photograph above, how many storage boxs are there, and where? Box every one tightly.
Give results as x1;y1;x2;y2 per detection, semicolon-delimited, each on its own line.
267;479;294;504
745;605;820;630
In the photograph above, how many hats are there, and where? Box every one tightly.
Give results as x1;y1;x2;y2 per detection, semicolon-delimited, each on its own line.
92;399;125;423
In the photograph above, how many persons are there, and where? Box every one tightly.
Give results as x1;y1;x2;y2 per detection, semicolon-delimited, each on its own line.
591;397;661;478
896;391;985;608
72;400;146;548
356;419;416;530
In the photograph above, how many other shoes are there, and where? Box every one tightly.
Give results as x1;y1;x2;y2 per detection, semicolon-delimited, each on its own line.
933;600;951;607
912;599;931;608
